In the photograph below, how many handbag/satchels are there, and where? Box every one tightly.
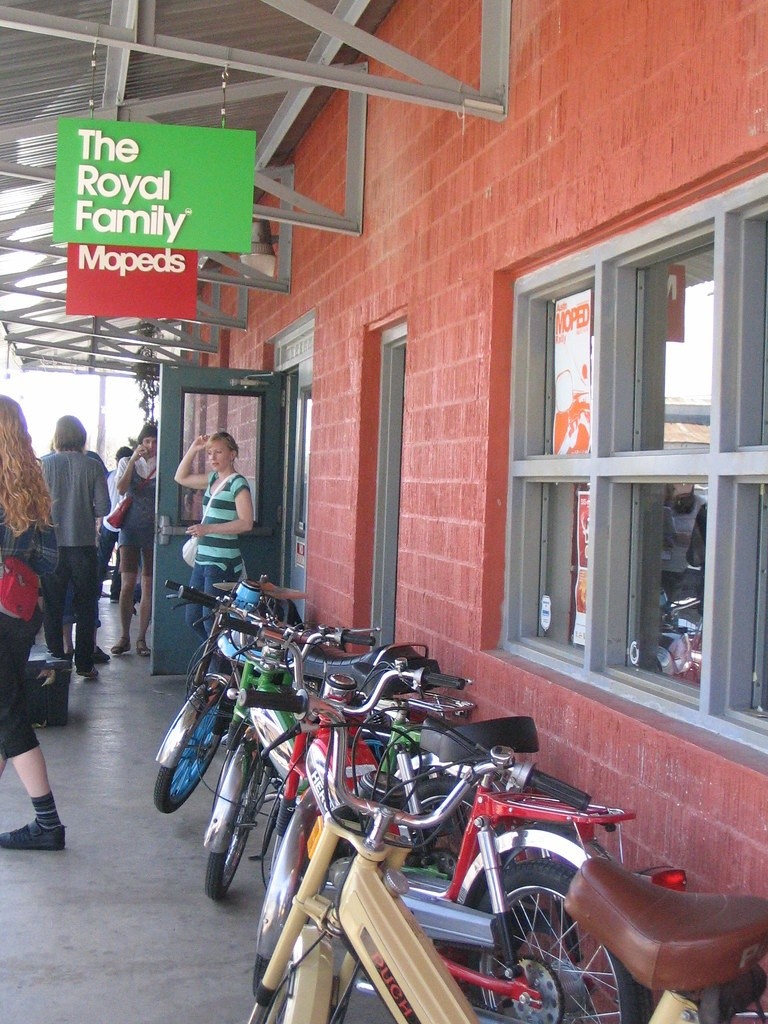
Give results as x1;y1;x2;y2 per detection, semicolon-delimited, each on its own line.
107;496;132;528
0;557;39;621
182;535;197;567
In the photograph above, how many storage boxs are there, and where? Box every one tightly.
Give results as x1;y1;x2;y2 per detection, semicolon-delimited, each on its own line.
17;642;73;726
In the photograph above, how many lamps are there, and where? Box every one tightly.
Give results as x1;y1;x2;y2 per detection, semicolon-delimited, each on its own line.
239;218;280;282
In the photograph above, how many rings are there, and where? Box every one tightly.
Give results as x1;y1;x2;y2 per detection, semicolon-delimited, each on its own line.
195;533;197;535
139;453;142;456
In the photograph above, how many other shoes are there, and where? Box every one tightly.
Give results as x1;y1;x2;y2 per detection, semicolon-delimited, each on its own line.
77;666;98;677
111;637;130;654
137;640;151;656
110;597;119;602
91;646;110;660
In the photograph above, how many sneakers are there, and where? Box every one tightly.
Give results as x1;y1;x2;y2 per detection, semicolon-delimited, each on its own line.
0;818;65;851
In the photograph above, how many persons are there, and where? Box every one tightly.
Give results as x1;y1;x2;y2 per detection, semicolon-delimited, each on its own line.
663;483;707;600
36;414;158;677
0;393;66;851
174;432;254;674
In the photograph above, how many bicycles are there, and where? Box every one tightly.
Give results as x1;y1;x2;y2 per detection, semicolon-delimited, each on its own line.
143;573;768;1024
